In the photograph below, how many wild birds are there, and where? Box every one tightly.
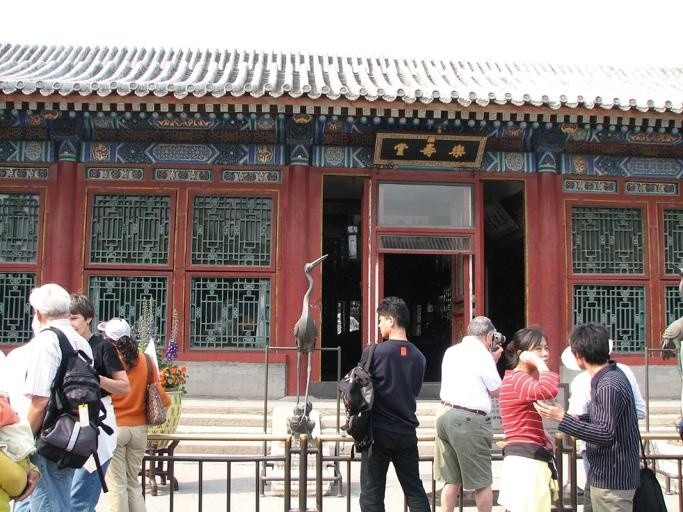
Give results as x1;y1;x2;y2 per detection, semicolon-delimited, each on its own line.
293;253;329;422
659;262;683;417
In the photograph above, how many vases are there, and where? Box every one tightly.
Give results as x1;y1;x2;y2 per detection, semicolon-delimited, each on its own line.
145;392;183;450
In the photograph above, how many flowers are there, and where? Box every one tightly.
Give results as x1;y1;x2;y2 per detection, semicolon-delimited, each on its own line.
120;288;189;398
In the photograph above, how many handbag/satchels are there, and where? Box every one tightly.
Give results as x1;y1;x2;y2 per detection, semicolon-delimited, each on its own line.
143;352;166;425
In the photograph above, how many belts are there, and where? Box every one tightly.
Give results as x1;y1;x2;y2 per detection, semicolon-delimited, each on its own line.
441;401;486;416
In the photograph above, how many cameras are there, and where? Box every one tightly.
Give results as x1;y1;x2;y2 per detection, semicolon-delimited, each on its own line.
492;331;507;348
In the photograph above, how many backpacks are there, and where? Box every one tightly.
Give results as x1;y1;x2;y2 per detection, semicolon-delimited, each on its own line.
34;326;113;492
338;342;377;464
633;468;667;512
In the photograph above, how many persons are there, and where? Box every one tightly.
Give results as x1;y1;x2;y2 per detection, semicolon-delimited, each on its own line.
1;282;172;511
560;334;648;477
433;316;504;511
497;326;560;511
533;322;644;511
358;297;430;512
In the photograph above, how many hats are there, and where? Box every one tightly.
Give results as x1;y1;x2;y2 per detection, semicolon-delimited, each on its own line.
561;339;613;371
97;317;130;341
